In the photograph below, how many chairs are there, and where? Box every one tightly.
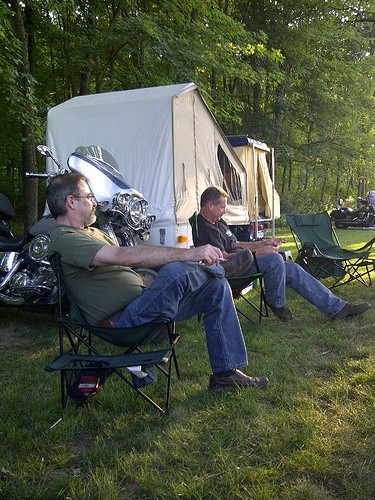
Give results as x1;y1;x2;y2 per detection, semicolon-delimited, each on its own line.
188;211;269;326
285;211;375;290
44;253;183;417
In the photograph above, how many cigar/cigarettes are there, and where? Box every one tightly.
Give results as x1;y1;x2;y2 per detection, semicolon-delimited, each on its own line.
218;257;227;262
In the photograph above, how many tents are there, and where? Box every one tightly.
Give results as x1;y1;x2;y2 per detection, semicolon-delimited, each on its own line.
225;132;281;220
47;83;249;224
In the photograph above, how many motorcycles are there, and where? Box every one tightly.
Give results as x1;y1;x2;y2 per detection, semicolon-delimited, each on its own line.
0;145;164;315
330;191;375;229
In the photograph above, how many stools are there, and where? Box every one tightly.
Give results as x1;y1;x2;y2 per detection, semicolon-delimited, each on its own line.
278;250;293;261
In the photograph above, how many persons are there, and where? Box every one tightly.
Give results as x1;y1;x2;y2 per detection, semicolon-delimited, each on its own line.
47;172;271;389
190;186;371;325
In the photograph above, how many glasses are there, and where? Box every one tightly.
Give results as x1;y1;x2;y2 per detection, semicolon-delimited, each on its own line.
69;192;95;202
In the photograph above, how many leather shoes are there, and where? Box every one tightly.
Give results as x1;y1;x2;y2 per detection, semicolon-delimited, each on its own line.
265;301;292;322
209;369;269;390
219;249;254;279
332;301;372;320
